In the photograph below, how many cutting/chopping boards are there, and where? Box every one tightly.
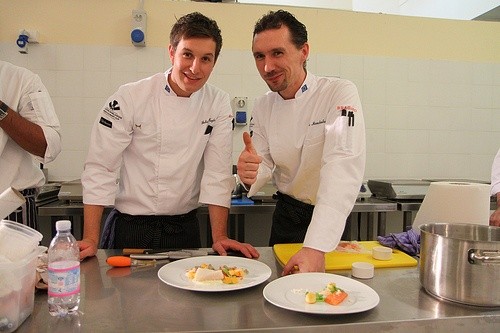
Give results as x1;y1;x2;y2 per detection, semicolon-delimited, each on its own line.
272;242;418;273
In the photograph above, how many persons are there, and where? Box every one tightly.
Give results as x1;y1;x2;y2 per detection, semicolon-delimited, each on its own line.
489;148;500;227
238;9;367;276
1;59;64;231
56;12;260;263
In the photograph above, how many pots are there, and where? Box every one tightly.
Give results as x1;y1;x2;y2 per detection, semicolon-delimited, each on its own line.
418;222;500;308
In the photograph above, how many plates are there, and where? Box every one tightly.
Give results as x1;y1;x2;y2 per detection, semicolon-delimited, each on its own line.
263;300;379;326
157;255;272;292
263;273;380;315
156;280;265;307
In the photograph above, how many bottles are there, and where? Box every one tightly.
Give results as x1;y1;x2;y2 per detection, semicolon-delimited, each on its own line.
47;220;80;317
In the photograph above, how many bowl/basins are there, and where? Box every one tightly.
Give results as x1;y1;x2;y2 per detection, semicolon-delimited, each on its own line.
372;246;393;260
352;262;375;279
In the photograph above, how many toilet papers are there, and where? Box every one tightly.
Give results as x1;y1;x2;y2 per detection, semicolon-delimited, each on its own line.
411;182;491;228
0;186;26;220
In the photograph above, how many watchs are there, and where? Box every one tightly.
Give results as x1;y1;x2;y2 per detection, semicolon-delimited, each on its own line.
0;101;8;121
